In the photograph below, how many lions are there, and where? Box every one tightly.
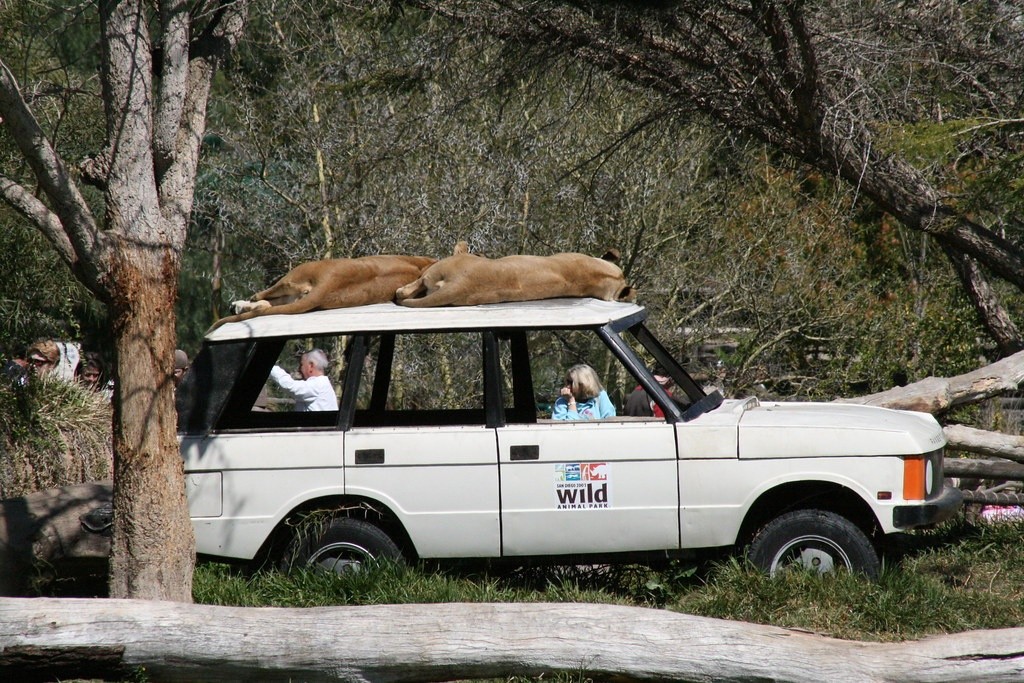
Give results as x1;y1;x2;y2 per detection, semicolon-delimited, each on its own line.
200;239;637;337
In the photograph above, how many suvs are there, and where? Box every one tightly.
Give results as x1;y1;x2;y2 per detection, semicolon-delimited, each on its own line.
179;296;962;605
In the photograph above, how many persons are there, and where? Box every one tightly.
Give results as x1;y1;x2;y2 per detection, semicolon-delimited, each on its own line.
11;336;189;409
551;365;616;420
622;361;675;417
268;350;339;412
